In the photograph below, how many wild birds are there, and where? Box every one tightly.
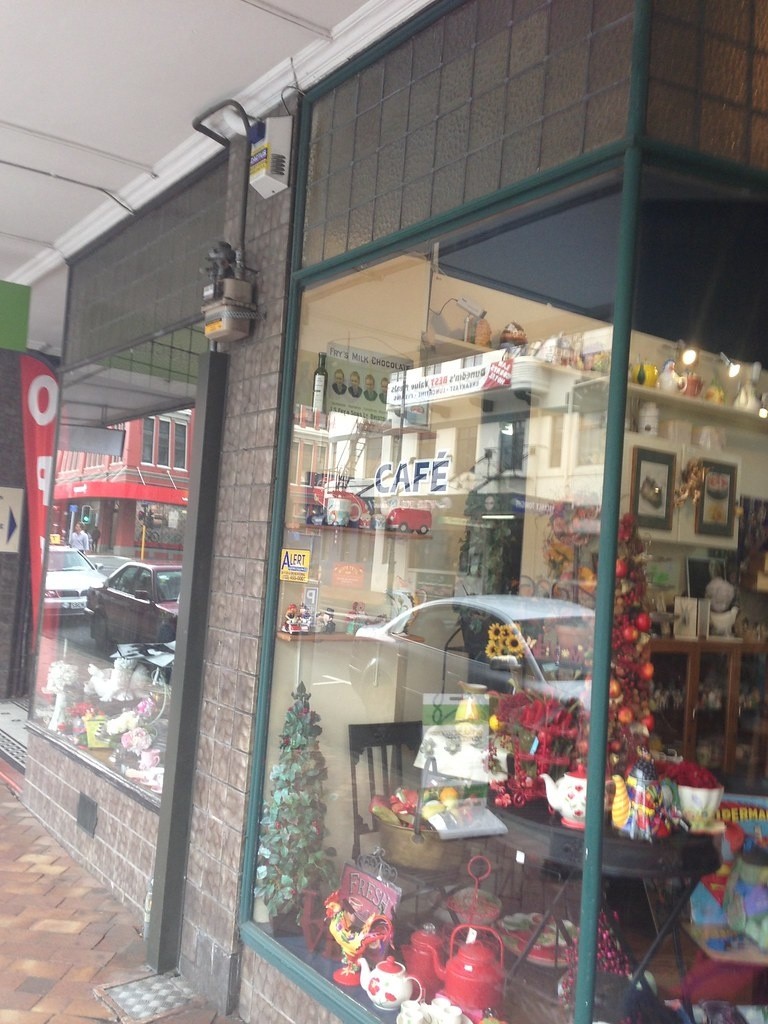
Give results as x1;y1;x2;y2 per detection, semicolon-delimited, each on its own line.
709;606;740;637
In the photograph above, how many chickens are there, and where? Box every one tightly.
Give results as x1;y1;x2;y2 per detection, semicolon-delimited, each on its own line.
656;359;688;396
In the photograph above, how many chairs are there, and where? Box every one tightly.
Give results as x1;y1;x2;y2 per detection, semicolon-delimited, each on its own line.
349;721;482;927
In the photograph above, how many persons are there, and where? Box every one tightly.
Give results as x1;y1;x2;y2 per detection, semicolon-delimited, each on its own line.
282;602;387;634
332;368;391;404
70;521;101;557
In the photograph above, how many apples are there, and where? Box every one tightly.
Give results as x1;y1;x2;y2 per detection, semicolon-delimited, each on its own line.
573;513;655;755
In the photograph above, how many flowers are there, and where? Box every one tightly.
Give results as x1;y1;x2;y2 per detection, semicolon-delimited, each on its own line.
253;682;338;928
485;622;538;660
57;701;106;749
106;697;160;757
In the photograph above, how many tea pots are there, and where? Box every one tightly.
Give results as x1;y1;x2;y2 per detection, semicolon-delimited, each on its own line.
540;765;615;827
359;957;422;1010
612;753;682;841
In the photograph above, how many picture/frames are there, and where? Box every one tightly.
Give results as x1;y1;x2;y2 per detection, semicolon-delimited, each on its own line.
630;445;677;530
694;457;737;538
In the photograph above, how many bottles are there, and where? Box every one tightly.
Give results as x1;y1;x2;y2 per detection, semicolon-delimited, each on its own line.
312;352;327;430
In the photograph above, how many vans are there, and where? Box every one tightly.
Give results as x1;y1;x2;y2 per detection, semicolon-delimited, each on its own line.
385;508;432;534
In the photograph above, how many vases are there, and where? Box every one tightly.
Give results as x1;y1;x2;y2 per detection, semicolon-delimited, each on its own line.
268;910;303;938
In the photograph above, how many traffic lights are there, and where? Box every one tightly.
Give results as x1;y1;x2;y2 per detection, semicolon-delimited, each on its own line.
80;505;90;525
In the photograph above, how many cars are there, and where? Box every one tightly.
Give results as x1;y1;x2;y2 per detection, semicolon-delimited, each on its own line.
41;545;111;629
344;594;650;748
87;562;195;662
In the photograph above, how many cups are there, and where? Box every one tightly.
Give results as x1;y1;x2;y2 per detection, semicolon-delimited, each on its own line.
402;1012;423;1024
444;1006;463;1024
401;1001;420;1012
639;402;659;435
327;498;361;526
633;362;658;386
659;359;688;393
432;999;450;1011
141;750;160;767
674;785;724;827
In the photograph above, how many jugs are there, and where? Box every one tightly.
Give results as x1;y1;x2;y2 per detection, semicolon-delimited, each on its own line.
426;923;503;1020
455;680;491;737
402;922;448;1002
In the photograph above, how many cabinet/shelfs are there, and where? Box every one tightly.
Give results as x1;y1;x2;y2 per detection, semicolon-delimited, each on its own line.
570;428;742;550
555;623;768;780
276;411;440;643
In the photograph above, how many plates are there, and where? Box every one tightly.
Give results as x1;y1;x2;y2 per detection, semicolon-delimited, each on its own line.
428;885;502;925
396;1005;473;1024
495;913;576;967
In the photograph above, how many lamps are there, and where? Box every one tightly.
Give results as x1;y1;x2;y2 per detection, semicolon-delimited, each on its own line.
718;351;741;377
673;339;697;365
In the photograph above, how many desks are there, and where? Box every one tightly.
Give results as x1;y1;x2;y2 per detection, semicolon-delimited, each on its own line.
487;802;723;1024
